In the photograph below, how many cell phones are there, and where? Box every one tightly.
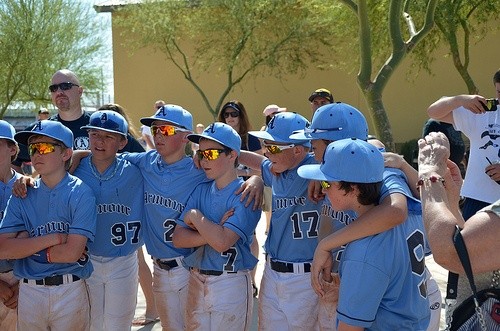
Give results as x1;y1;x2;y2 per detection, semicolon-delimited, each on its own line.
477;98;498;111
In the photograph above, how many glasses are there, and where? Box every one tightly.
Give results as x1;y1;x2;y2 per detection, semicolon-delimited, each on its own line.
48;82;80;91
195;150;229;160
262;140;299;154
150;125;187;136
317;180;331;190
223;112;238;118
27;143;64;156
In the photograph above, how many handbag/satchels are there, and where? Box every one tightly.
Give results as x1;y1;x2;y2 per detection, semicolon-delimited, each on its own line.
438;225;500;331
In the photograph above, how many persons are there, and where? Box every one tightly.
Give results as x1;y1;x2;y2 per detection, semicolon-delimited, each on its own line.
218;101;262;182
0;120;23;331
297;137;431;331
192;124;204;157
142;100;165;153
258;105;287;234
0;120;95;331
237;111;322;331
67;105;264;331
417;116;500;331
289;102;442;331
47;70;92;151
98;103;147;153
11;110;144;331
173;122;261;331
425;69;500;222
38;108;51;121
308;88;334;113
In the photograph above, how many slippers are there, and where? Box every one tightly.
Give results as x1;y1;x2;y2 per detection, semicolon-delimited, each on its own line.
131;315;160;327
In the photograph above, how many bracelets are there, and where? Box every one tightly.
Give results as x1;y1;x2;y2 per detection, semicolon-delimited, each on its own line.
47;246;53;264
416;175;446;193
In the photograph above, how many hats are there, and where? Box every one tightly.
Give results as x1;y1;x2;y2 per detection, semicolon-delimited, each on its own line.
297;137;384;183
80;110;130;136
187;122;242;157
309;89;335;102
139;104;193;132
247;112;311;148
14;120;75;148
289;101;368;142
0;120;17;144
263;104;287;117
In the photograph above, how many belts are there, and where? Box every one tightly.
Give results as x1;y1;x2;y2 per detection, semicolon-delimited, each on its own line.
265;253;311;273
23;274;80;286
155;259;179;270
189;266;237;276
